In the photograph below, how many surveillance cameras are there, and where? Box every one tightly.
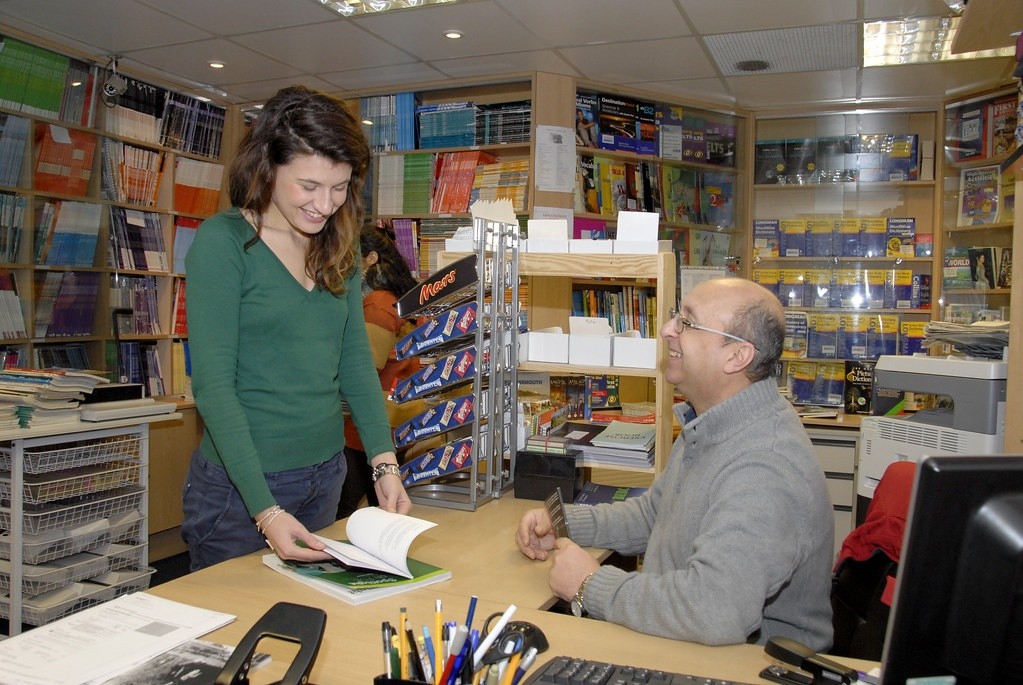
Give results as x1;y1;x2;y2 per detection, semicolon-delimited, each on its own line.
103;75;128;97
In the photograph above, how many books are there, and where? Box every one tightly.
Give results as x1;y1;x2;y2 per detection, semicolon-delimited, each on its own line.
108;639;272;685
0;36;532;431
309;506;439;580
572;88;1022;419
261;539;452;605
516;373;658;504
543;487;572;540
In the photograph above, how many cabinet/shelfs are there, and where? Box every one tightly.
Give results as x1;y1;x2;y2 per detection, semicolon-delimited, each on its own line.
936;92;1023;358
746;107;938;411
442;252;677;492
0;23;243;409
570;95;748;419
1;414;188;635
390;218;523;511
313;73;539;439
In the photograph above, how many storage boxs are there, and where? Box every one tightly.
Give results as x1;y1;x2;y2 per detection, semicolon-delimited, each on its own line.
755;134;933;415
445;210;675;368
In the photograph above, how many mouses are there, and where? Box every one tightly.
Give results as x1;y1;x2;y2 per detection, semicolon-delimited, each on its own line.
498;620;550;662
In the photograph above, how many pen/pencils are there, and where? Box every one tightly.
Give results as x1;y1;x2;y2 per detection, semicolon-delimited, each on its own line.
382;608;427;682
422;596;478;685
499;647;538;685
457;605;517;685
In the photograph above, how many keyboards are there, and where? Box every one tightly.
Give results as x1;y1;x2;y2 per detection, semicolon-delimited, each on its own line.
522;654;759;685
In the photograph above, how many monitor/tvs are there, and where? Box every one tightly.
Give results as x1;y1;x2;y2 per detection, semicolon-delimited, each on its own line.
878;452;1023;685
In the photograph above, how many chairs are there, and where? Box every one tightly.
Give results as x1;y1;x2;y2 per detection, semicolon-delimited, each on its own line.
811;462;918;662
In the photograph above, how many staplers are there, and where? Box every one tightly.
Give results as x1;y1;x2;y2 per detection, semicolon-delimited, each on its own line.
759;637;859;685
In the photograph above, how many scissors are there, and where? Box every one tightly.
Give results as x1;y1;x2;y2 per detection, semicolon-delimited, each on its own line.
473;611;525;674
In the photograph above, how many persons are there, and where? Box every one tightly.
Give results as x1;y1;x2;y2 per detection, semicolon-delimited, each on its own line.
515;276;834;649
975;254;989;289
337;220;419;522
577;110;599;149
181;84;411;573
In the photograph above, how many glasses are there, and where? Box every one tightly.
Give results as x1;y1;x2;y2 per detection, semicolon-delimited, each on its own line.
668;307;763;353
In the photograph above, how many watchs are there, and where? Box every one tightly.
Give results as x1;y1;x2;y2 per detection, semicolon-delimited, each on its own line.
571;573;593;617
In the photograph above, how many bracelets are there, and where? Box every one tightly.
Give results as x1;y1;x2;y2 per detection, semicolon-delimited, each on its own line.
372;463;401;481
256;505;285;534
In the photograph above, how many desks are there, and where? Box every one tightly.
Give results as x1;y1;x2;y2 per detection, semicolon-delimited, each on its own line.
0;495;880;685
